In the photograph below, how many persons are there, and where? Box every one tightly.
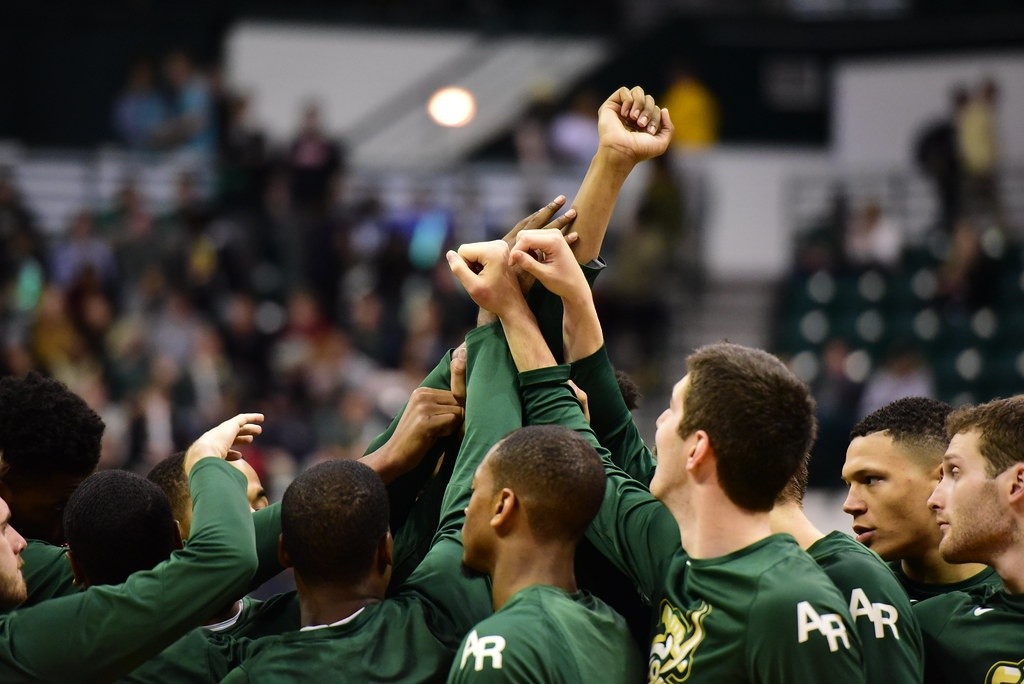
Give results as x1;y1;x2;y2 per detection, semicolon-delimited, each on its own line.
910;394;1024;684
0;413;264;684
0;370;106;611
842;396;995;607
0;75;1024;499
63;85;924;684
